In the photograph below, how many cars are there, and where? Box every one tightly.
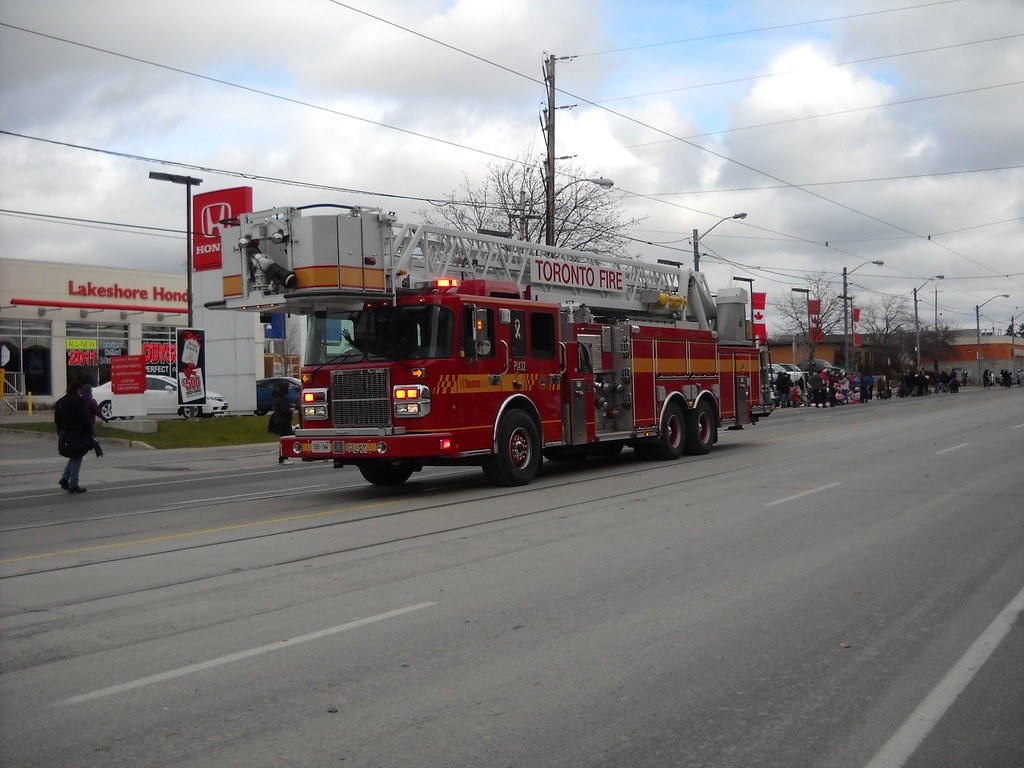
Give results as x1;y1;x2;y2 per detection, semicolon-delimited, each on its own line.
766;363;816;386
254;377;301;416
796;358;846;377
92;374;228;422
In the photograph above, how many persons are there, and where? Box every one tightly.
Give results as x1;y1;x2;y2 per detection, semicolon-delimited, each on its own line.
81;384;108;428
272;381;294;465
341;328;374;353
774;368;1024;408
53;382;104;494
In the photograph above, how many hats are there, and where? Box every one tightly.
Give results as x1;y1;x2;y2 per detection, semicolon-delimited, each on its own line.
277;381;289;394
82;383;92;393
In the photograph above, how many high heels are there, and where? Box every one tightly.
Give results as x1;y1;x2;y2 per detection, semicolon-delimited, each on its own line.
59;477;71;490
69;485;87;494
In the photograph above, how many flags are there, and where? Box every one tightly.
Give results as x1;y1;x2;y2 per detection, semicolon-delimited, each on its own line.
753;294;767;346
808;300;823;341
853;307;862;346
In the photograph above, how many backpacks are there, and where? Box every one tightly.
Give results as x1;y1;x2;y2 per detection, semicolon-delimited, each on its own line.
268;406;281;435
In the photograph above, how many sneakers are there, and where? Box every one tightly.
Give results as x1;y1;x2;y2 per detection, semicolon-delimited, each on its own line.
277;458;294;465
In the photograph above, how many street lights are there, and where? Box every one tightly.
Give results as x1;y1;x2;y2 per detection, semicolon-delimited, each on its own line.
147;170;203;417
733;276;754;347
842;258;884;376
912;274;945;375
545;177;614;258
691;212;748;273
837;295;855;374
506;213;541;242
791;287;811;350
975;294;1010;389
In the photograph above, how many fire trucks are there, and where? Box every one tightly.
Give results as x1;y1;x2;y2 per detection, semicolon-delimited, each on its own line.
203;203;779;488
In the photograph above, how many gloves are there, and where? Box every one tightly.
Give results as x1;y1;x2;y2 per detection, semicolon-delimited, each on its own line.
104;418;110;423
